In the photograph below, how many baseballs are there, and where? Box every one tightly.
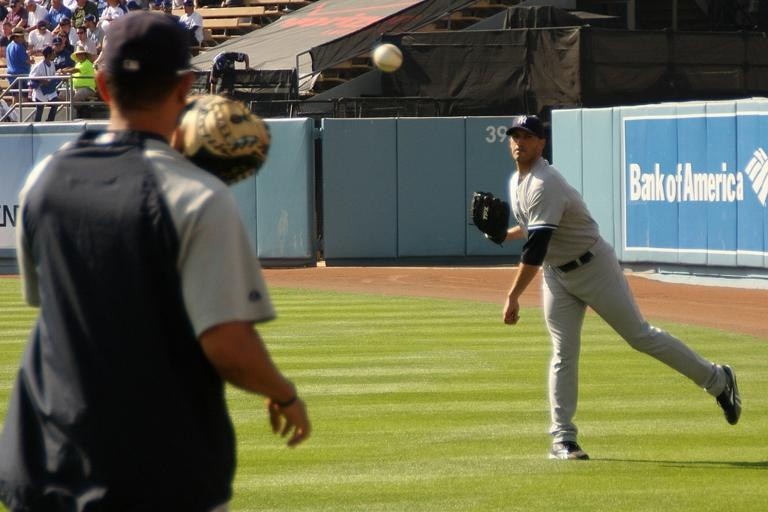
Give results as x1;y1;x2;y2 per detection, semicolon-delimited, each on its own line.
374;44;403;72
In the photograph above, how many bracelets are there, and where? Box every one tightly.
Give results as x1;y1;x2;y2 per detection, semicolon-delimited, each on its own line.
272;395;298;411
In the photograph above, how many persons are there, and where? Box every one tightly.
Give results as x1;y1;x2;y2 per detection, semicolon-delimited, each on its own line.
471;115;742;460
0;10;312;512
0;0;219;122
210;50;249;96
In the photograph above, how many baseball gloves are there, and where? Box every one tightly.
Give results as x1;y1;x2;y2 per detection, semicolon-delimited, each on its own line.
171;94;269;187
472;191;509;248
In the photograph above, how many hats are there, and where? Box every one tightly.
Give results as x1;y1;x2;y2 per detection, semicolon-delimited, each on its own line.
8;27;25;41
215;55;225;70
506;114;544;138
73;46;87;54
52;37;62;45
102;10;193;75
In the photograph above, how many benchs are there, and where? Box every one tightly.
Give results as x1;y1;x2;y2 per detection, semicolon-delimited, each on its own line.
0;1;311;106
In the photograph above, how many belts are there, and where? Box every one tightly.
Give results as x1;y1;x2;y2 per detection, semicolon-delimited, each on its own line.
558;251;594;272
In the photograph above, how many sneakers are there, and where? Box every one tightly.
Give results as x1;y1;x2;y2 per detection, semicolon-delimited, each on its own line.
716;364;742;425
549;440;589;460
